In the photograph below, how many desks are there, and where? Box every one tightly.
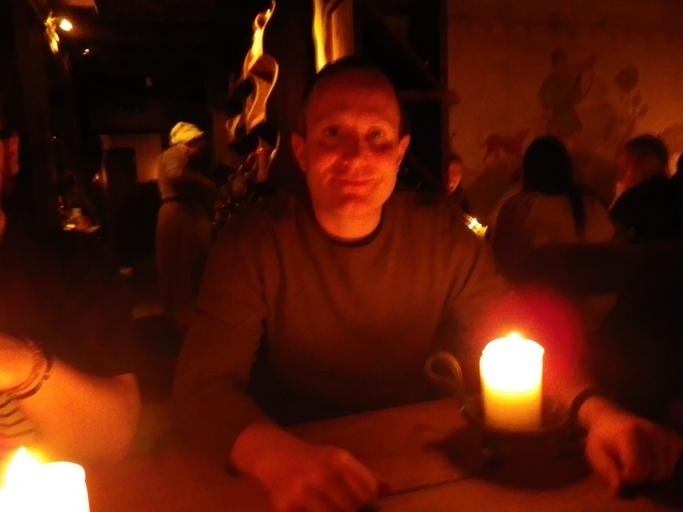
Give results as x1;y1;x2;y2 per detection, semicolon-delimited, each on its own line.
0;394;665;511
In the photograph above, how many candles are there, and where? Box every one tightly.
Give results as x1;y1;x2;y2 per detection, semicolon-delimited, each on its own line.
480;329;547;433
0;446;92;511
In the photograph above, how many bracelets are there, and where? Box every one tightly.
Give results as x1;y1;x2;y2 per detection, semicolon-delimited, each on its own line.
566;387;619;432
2;333;56;403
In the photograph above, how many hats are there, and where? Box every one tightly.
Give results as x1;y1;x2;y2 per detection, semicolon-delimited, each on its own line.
170;121;205;144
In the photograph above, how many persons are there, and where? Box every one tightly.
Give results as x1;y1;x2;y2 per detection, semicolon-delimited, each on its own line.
610;137;683;246
163;51;682;509
447;150;470;214
492;134;618;245
152;121;228;331
0;110;145;468
